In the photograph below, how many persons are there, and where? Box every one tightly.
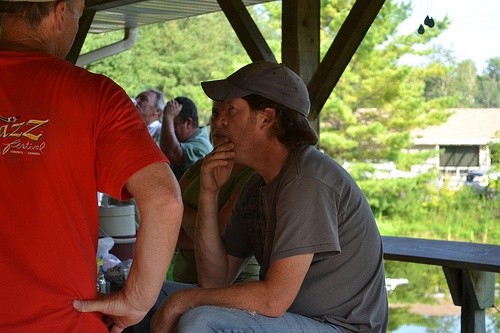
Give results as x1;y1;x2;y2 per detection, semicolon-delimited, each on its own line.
159;96;214;183
172;100;261;285
101;90;162;226
124;61;389;333
0;0;184;333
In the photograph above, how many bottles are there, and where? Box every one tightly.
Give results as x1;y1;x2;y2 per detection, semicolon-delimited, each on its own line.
97;274;106;294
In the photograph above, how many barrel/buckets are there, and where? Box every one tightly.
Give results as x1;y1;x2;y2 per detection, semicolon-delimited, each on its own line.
98;205;136;238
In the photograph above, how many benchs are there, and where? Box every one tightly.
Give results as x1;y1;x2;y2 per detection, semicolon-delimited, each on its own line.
380;235;500;333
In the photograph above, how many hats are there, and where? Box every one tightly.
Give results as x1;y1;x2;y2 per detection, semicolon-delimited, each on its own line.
201;62;310;117
